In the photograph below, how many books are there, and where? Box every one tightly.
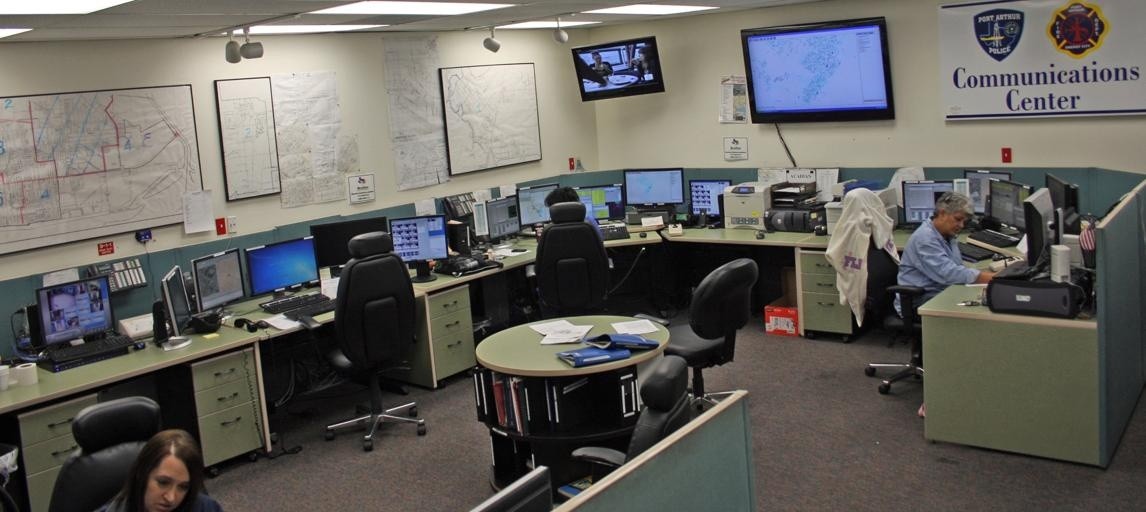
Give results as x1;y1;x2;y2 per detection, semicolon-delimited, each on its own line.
556;333;660;368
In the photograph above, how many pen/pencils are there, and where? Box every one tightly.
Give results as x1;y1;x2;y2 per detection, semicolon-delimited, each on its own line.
1004;260;1007;267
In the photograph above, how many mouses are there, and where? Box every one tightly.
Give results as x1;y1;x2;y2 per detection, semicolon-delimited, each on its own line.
134;342;146;349
639;232;647;237
756;233;764;239
256;320;268;328
991;253;1004;261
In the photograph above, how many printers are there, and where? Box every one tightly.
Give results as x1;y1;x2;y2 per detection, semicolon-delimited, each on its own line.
723;180;781;230
823;178;899;236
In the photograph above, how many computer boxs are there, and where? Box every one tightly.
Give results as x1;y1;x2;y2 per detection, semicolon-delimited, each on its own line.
446;221;471;257
764;200;830;232
985;267;1092;319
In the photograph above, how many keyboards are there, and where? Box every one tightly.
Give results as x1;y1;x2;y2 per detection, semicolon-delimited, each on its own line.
257;290;330;314
48;334;134;363
967;229;1020;248
282;299;336;322
598;223;630;240
956;240;994;263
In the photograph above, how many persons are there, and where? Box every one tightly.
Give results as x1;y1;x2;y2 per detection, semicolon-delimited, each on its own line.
632;47;654;74
544;186;603;242
588;52;614;75
93;429;224;512
893;191;999;324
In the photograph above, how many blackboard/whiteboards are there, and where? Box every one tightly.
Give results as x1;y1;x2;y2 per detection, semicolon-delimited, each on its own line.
0;83;204;254
438;63;542;176
214;78;280;202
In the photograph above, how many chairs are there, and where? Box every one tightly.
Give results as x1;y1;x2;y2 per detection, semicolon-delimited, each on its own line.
295;229;430;454
664;255;762;418
514;200;611;321
47;394;165;508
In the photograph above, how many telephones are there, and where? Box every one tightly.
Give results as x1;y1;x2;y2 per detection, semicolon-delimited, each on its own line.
441;195;466;220
87;261;119;295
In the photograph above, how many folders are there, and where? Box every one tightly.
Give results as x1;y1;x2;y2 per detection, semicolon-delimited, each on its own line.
555;333;659;367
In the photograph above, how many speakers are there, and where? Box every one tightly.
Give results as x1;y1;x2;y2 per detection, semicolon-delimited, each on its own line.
153;299;168;347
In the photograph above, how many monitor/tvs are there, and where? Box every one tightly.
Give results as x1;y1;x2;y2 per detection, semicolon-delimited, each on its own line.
739;17;896;124
571;34;666;103
516;167;732;234
35;275;115;349
160;214;450;336
904;170;1081;268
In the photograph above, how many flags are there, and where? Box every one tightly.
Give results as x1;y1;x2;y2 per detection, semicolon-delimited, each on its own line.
1080;219;1098;252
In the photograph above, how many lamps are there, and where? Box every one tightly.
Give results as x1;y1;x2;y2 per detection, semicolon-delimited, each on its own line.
192;12;304;64
464;11;576;53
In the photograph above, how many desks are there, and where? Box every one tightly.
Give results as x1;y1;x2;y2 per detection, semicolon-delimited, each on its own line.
198;259;480;453
430;216;662;322
466;313;670;497
0;320;271;511
661;198;858;343
873;182;1145;485
